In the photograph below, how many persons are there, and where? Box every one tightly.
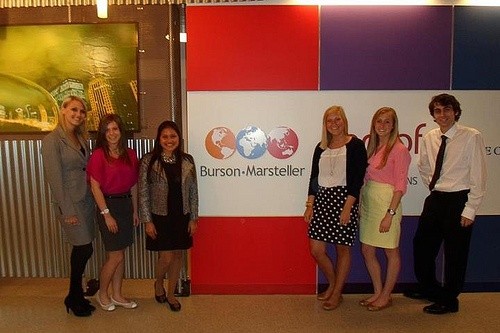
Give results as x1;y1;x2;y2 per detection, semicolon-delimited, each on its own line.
139;121;199;311
86;114;140;311
359;107;411;311
42;95;96;316
304;106;368;310
404;94;486;315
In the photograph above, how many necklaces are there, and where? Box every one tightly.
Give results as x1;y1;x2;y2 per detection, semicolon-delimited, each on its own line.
164;156;172;162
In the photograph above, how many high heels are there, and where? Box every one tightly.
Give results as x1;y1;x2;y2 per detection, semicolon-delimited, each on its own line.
64;294;96;317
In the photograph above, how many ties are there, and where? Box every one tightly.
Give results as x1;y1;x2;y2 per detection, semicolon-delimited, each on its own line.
428;134;448;191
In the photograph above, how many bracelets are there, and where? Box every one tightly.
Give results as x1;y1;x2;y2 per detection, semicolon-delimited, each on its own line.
100;208;109;214
306;201;314;207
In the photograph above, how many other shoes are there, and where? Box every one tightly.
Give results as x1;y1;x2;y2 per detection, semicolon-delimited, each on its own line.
359;294;392;311
404;281;440;300
423;298;460;314
110;295;137;309
154;281;166;303
322;295;343;310
166;297;181;311
317;292;333;301
95;290;116;311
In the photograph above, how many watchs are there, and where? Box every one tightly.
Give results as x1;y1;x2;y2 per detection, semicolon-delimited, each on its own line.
387;209;396;215
